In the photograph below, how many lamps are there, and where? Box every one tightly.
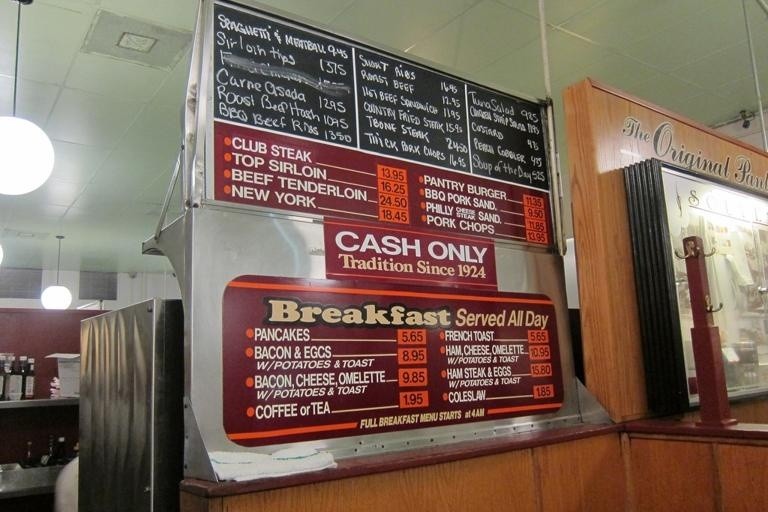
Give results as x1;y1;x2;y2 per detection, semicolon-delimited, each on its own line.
40;235;73;310
0;0;55;196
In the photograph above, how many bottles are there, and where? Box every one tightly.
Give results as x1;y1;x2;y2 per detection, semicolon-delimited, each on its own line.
23;441;34;468
45;434;57;466
0;353;36;402
57;437;67;465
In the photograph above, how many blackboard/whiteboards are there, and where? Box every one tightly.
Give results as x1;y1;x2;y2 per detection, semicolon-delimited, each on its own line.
211;2;551;192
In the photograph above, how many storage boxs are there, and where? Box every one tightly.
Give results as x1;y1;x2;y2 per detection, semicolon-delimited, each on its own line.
45;353;80;398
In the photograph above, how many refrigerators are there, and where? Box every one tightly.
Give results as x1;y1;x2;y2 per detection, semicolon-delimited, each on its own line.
72;297;186;512
